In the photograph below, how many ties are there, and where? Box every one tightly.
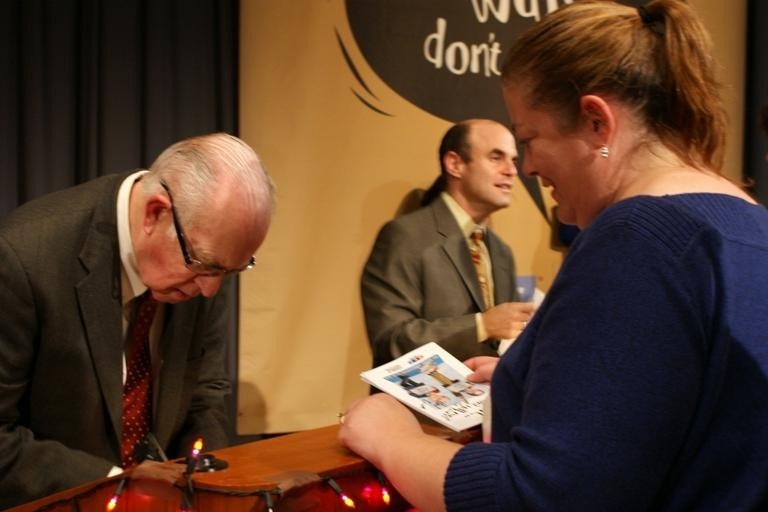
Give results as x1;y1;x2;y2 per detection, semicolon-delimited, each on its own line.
121;293;159;471
467;230;493;309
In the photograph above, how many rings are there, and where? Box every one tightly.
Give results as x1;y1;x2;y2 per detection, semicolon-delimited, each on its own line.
338;411;348;426
523;321;527;328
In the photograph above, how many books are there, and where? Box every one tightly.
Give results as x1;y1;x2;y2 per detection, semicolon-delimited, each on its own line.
360;339;493;433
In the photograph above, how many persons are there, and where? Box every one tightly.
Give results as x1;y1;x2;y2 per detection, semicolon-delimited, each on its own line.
339;1;768;512
359;116;536;396
0;130;275;501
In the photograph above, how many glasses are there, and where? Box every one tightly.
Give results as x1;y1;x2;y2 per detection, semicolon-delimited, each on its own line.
160;180;256;277
